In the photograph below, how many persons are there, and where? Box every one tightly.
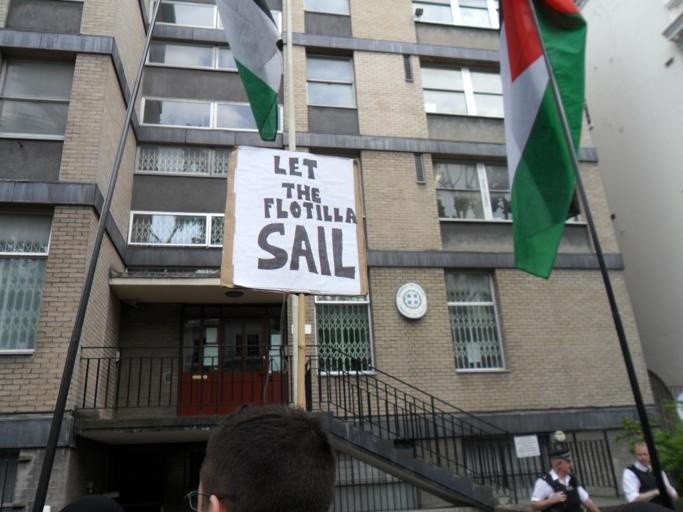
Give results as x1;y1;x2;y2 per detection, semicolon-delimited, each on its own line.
196;401;337;512
621;440;680;504
530;447;602;512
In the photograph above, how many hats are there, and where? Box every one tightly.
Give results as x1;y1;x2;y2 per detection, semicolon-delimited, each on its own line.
547;449;571;458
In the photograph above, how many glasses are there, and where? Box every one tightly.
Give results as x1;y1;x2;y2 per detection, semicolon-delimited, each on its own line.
187;491;224;512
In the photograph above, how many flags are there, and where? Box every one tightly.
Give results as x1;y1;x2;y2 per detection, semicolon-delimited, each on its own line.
215;0;284;143
498;0;590;284
